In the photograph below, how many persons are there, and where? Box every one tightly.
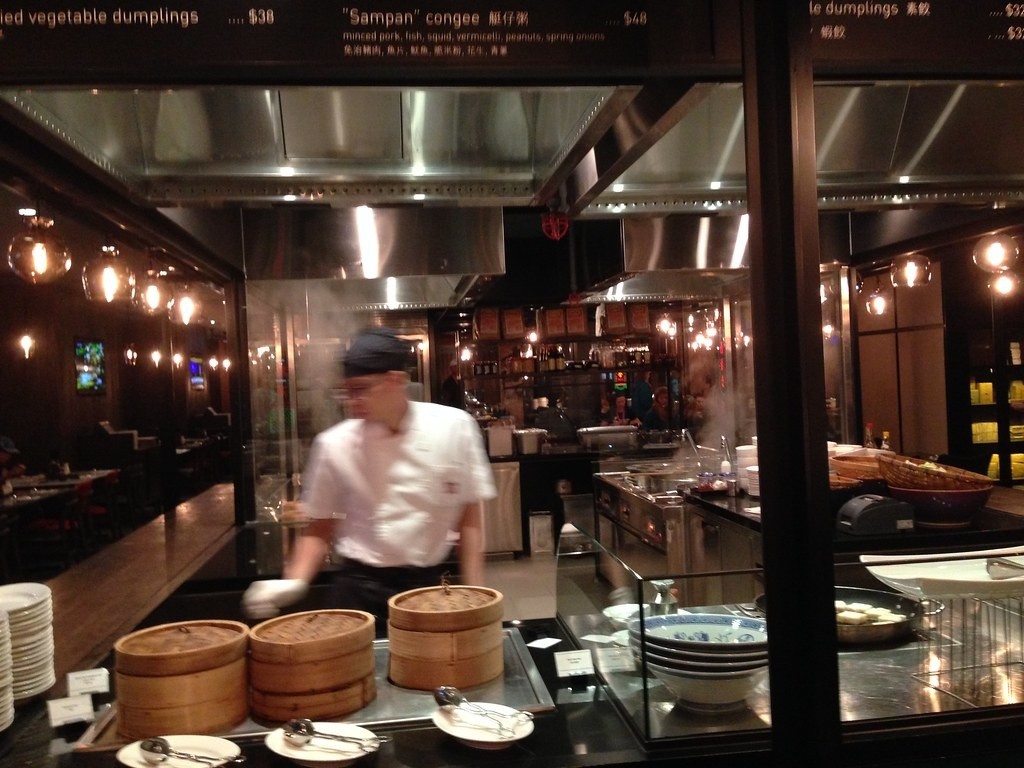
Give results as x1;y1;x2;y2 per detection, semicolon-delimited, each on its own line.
600;371;757;433
0;435;26;483
242;327;498;637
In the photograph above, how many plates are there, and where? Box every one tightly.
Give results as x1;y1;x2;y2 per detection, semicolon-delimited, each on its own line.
860;545;1024;598
264;722;382;767
115;734;241;767
0;582;56;732
430;701;535;750
602;603;690;647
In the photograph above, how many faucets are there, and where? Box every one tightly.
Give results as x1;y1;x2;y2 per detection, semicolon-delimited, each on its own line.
721;435;733;465
681;428;704;467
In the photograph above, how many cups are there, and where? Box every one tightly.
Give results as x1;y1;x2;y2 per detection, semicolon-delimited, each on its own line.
639;428;682;443
735;437;761;498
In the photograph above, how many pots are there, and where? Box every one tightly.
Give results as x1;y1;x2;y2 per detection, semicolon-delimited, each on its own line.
735;585;945;644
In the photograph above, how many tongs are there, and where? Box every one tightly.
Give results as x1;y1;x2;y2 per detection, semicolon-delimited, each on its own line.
680;429;735;476
433;686;536;737
282;718;393;753
140;737;247;768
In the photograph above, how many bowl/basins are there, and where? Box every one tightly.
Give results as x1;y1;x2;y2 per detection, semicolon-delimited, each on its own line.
627;613;769;714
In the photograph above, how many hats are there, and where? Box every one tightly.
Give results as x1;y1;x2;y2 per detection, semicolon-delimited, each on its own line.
0;437;20;454
342;328;411;379
654;385;668;396
450;359;457;366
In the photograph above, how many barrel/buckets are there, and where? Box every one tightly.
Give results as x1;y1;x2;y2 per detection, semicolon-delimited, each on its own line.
514;427;549;453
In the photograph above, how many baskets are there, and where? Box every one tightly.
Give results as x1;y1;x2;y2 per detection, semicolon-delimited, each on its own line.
830;455;884;479
878;453;994;490
829;476;863;490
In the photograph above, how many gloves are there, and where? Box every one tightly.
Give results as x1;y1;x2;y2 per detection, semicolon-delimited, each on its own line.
241;579;310;619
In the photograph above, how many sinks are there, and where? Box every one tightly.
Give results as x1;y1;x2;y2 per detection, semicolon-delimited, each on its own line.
593;471;698;509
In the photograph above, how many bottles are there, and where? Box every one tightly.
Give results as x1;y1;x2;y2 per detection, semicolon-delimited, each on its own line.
863;424;894;451
697;472;740;496
510;339;652;373
649;578;678;617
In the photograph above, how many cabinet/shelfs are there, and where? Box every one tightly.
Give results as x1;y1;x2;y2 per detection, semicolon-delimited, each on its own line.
461;329;674;433
